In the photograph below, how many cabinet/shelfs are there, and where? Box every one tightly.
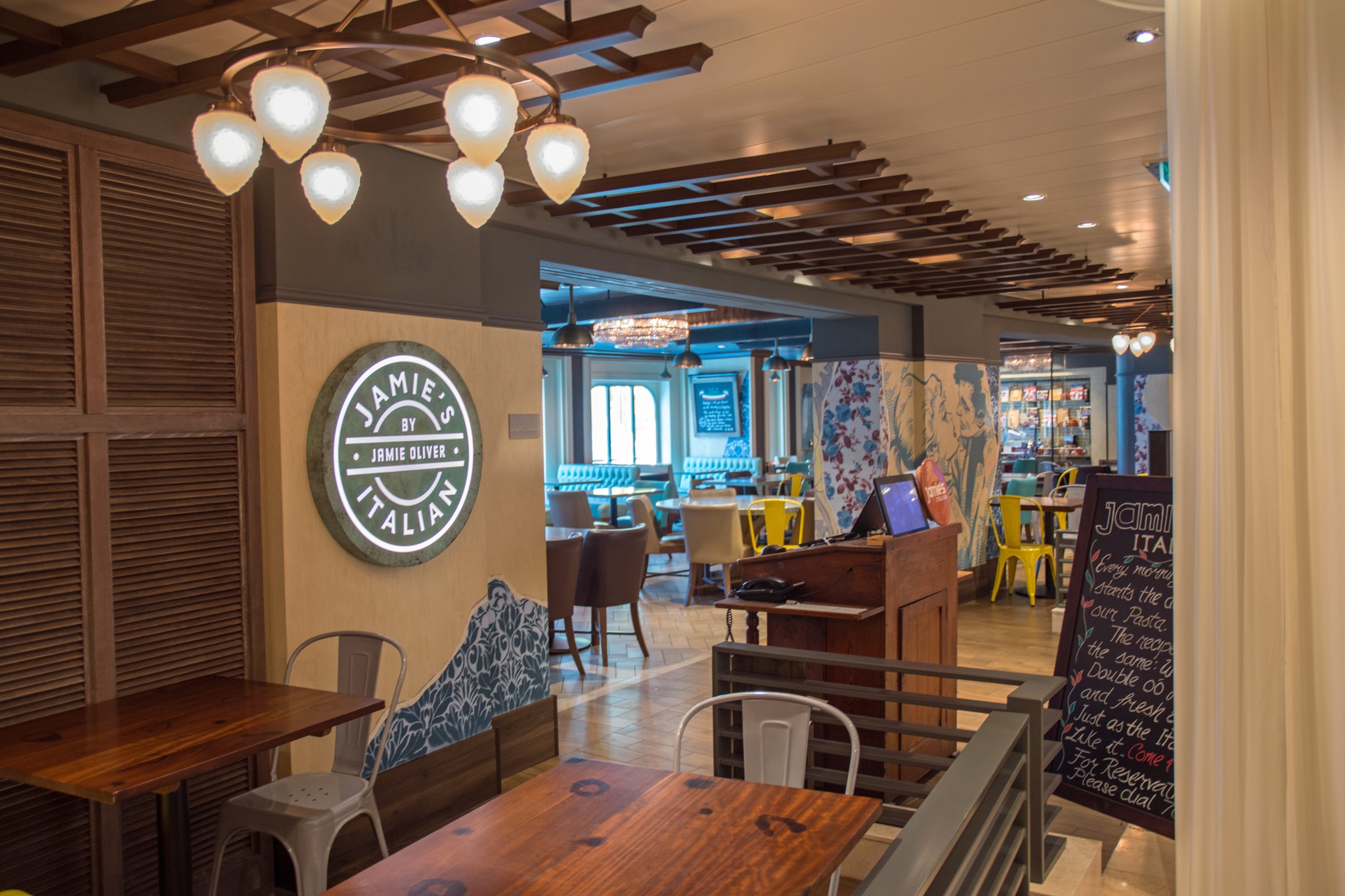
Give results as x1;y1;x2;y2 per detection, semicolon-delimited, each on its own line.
998;376;1092;465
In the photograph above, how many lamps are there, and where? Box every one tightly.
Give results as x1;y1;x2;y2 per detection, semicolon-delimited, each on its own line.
997;278;1174;359
0;0;718;232
547;283;814;382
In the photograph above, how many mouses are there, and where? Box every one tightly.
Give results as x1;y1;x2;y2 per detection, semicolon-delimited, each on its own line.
761;544;786;555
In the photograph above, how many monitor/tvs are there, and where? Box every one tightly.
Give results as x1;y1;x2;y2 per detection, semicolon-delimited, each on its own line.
873;474;929;537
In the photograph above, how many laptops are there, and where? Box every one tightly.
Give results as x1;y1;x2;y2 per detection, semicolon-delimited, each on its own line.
799;488;885;547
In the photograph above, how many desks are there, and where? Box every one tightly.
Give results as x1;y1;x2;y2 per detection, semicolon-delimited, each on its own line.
0;675;387;896
702;473;814;539
584;486;664;528
544;479;603;490
546;526;600;652
314;757;883;896
655;495;805;594
1054;465;1073;485
1000;472;1061;543
672;469;729;490
1001;459;1016;471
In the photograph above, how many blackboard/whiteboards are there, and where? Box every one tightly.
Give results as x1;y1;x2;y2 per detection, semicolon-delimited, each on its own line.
690;378;742;438
1044;472;1173;839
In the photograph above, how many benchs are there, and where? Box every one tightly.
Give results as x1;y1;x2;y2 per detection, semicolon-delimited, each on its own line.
637;464;679;498
557;463;640;523
678;457;762;495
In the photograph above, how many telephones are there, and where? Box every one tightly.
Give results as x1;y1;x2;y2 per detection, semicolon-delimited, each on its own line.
736;576;804;603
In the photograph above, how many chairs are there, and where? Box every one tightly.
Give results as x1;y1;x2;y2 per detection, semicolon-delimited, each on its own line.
207;630;409;896
674;690;861;896
545;456;817;676
987;456;1086;606
